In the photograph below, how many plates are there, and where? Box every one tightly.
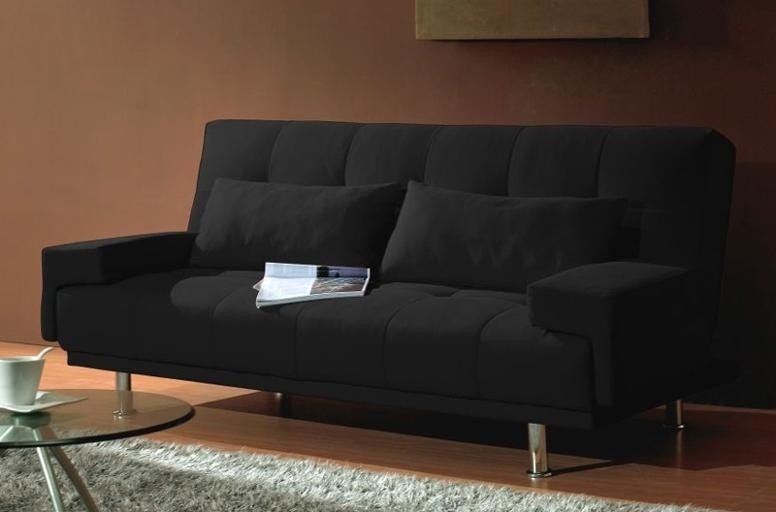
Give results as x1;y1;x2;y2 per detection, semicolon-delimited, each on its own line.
0;389;90;413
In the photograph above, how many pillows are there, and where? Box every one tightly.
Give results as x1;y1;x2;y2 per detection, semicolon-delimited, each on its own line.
376;175;628;291
190;173;401;279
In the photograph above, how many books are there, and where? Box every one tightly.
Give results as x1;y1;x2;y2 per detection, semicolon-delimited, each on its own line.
252;261;370;309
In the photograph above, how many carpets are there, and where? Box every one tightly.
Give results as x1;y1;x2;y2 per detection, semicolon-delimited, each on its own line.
0;425;726;512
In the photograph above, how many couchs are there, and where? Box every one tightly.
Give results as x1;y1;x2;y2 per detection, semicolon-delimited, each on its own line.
36;117;741;477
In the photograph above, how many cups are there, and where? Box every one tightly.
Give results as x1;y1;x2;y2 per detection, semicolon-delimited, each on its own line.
0;355;45;405
